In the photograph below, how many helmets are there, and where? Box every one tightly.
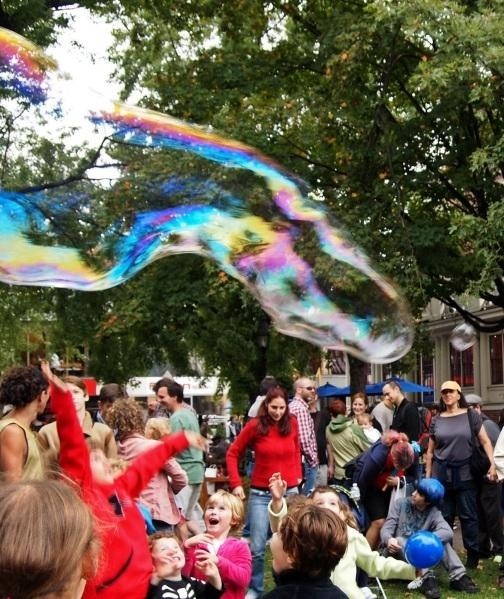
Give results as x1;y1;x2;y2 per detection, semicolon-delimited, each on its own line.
414;477;445;506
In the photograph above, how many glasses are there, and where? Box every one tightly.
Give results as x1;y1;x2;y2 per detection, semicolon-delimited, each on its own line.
299;387;315;391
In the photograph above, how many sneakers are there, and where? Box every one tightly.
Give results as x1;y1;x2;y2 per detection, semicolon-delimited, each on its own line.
498;576;504;589
418;576;441;598
245;589;262;598
450;574;480;593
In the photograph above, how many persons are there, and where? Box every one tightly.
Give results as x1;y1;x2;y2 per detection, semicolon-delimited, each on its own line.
0;361;504;599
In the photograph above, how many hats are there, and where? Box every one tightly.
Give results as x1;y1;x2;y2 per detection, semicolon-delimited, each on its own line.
464;393;483;407
440;381;461;393
